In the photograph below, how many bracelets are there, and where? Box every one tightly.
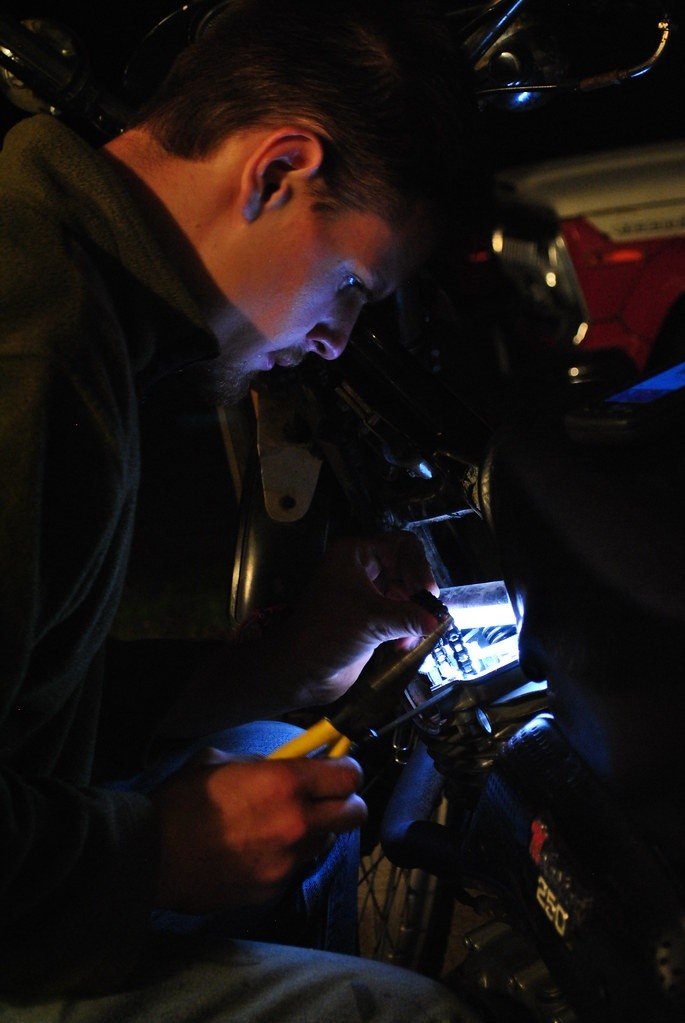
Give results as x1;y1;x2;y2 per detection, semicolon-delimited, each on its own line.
234;601;290;641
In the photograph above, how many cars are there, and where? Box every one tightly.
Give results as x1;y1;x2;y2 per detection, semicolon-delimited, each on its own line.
459;1;682;376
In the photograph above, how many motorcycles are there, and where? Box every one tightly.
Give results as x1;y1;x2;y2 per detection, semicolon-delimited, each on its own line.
1;0;684;1021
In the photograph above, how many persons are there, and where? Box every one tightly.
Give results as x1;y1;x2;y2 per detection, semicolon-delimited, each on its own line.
0;0;484;1023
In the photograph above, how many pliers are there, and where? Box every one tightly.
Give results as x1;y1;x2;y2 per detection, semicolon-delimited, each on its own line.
266;616;457;759
